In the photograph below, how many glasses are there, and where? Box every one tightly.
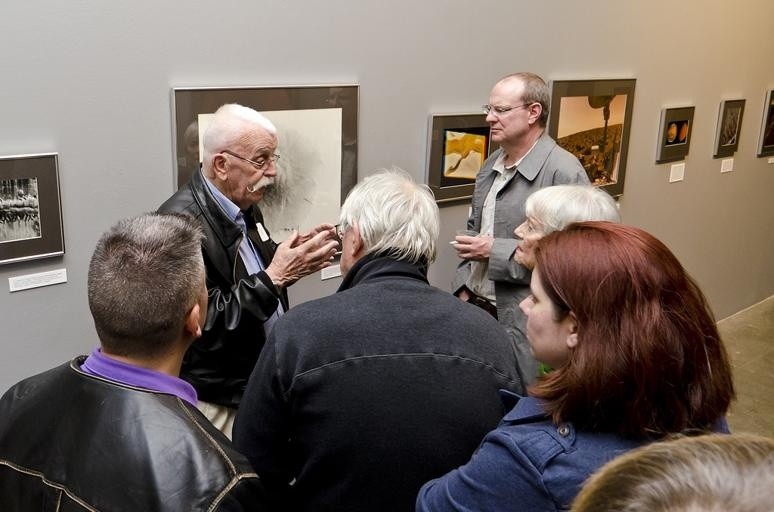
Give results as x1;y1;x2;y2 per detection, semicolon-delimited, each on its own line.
334;222;350;245
481;102;533;114
220;150;280;169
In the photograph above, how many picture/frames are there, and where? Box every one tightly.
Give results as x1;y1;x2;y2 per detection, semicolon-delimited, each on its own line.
713;98;747;158
424;111;493;204
755;89;774;155
0;151;66;266
655;104;695;162
546;75;638;199
169;82;360;256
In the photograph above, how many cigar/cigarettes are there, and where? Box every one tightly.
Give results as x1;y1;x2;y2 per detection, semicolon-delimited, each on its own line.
449;240;459;246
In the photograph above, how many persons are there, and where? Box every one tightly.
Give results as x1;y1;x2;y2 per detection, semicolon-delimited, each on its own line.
451;72;592;388
157;102;340;408
414;224;733;511
0;215;261;512
574;428;773;510
514;184;622;271
0;188;38;225
232;166;527;510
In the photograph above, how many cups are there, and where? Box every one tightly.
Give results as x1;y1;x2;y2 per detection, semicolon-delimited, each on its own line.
456;229;481;259
